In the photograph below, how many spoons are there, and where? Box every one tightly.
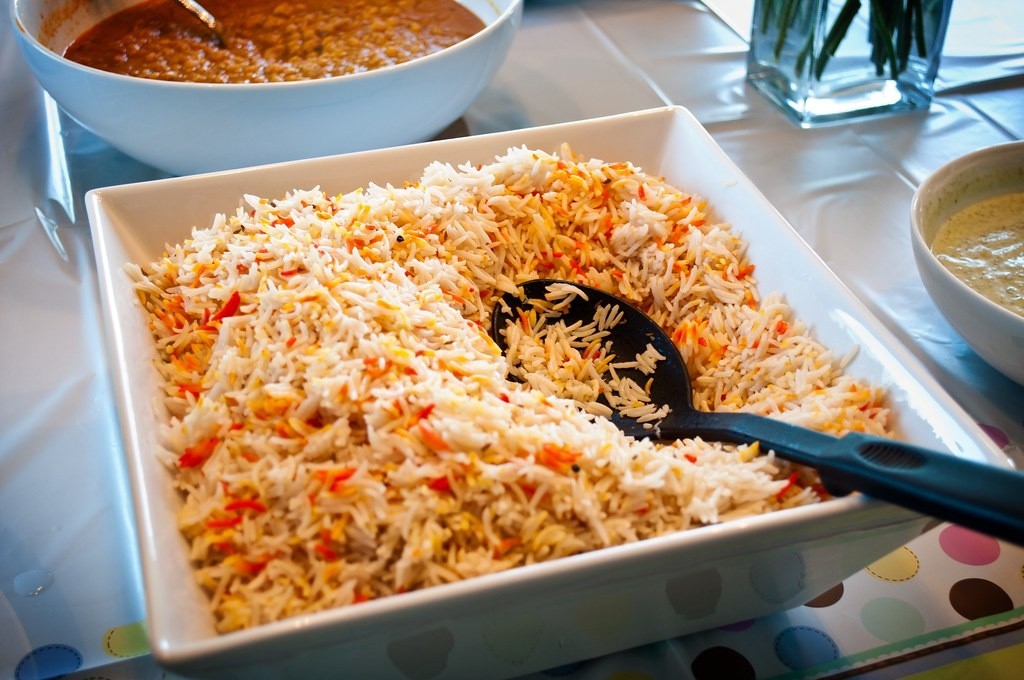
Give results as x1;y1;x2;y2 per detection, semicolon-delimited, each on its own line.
493;279;1024;549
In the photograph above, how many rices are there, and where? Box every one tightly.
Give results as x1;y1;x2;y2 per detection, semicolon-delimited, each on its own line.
123;141;899;635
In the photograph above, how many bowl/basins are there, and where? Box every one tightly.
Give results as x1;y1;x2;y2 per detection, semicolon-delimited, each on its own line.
85;104;1015;680
909;140;1024;387
9;0;525;177
